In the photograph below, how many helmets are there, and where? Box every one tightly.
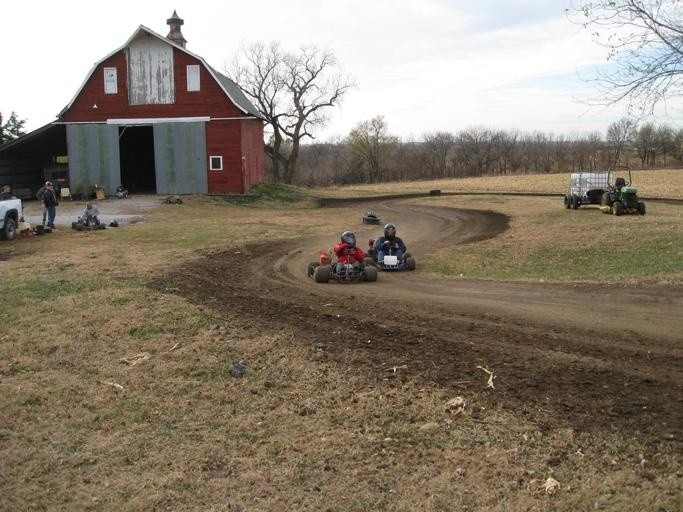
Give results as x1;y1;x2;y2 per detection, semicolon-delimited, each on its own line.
110;219;118;227
341;231;356;247
383;223;396;238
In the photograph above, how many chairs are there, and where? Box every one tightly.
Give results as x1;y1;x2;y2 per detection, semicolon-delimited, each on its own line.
60;188;72;202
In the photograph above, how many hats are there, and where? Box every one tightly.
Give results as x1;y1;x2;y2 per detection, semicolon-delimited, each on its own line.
47;182;54;186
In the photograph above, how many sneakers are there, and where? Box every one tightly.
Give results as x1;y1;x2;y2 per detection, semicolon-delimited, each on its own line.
340;267;346;280
397;256;403;265
352;266;359;279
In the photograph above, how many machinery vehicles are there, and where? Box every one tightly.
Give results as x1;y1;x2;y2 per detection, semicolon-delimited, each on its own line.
565;166;645;216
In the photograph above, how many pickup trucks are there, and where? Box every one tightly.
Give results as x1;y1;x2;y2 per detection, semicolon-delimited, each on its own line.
0;195;22;240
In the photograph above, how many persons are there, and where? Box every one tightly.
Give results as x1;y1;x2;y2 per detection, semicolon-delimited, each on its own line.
79;201;101;227
41;183;59;229
334;231;366;275
36;181;50;227
375;223;407;264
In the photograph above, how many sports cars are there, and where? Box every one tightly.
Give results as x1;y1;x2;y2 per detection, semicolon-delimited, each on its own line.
363;238;415;271
307;250;378;283
